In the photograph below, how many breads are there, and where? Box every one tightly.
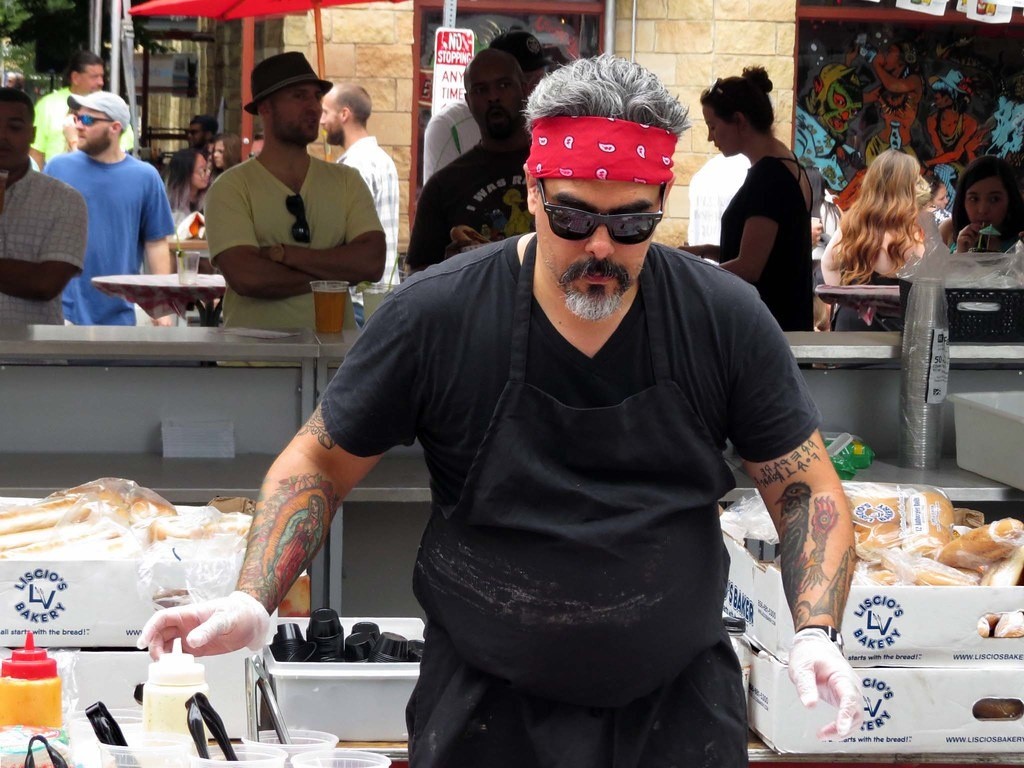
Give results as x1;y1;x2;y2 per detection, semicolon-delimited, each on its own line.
0;485;262;560
973;699;1023;719
844;490;1024;638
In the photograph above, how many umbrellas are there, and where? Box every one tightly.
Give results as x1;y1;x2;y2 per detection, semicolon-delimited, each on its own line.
128;0;407;161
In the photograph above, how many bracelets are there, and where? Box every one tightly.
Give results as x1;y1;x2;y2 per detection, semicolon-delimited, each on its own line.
798;625;844;655
69;141;76;147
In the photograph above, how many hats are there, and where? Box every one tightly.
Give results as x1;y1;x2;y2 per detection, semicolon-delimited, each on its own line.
67;90;131;133
488;30;552;73
244;51;333;115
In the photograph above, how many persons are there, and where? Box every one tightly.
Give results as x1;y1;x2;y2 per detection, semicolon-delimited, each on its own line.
405;48;536;272
321;84;399;325
0;85;88;324
939;155;1024;341
162;112;242;216
422;31;561;188
676;66;814;332
136;55;865;768
43;92;175;326
31;51;134;172
813;149;948;330
204;52;386;367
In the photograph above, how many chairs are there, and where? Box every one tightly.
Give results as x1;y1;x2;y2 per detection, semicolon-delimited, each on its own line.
816;284;900;332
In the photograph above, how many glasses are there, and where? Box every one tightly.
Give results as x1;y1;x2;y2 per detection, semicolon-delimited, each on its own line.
194;167;212;179
183;129;203;136
210;148;225;157
534;177;667;245
284;193;310;243
71;112;113;126
710;77;724;95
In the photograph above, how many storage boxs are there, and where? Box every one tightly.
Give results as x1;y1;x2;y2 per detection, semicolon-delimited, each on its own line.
946;391;1024;492
263;616;425;742
729;637;1024;753
725;529;1024;669
899;279;1024;343
0;651;149;712
278;575;311;616
0;558;243;647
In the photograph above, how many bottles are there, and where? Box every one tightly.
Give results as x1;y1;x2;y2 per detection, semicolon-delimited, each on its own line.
142;638;210;745
0;631;62;729
722;616;753;706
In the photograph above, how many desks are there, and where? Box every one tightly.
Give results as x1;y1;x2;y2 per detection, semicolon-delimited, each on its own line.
91;274;225;327
170;241;207;250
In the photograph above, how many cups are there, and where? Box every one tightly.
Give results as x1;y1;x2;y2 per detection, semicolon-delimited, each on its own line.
175;251;201;285
309;280;350;333
811;217;820;250
0;710;393;768
270;607;425;663
897;277;950;470
361;284;398;324
